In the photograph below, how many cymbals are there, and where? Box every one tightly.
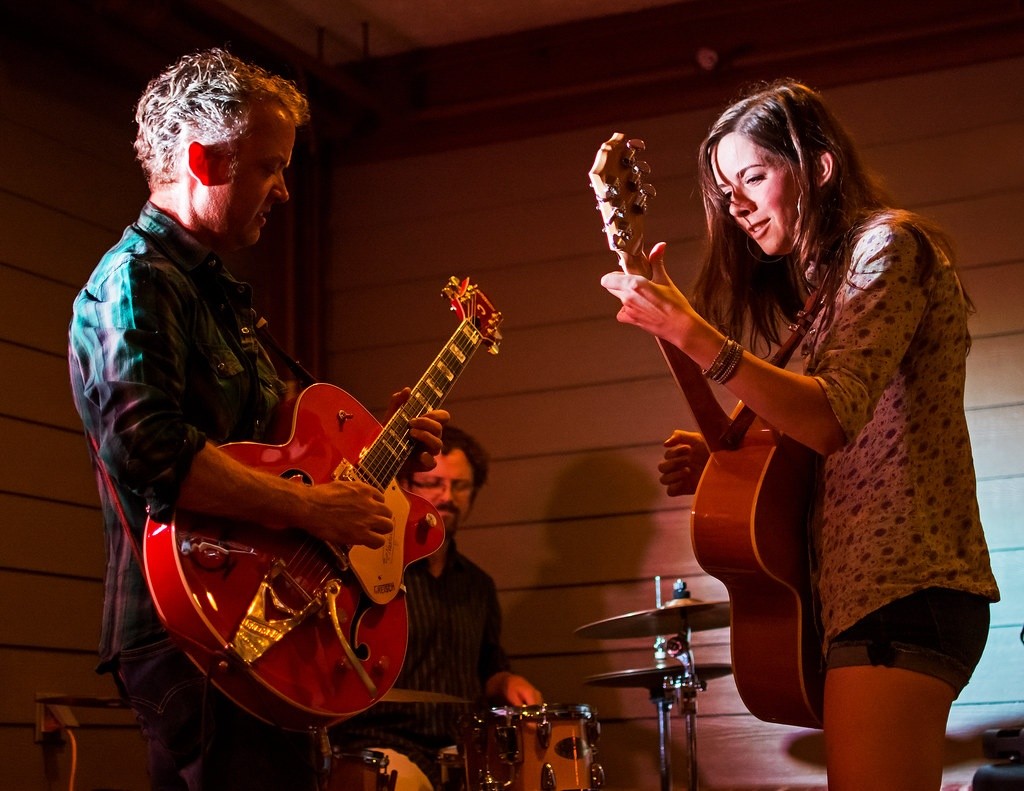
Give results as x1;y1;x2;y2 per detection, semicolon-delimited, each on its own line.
570;588;734;642
367;685;481;710
582;655;738;699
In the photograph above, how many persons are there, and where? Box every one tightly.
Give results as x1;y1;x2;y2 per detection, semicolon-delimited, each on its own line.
66;51;451;791
343;425;543;791
600;83;1001;791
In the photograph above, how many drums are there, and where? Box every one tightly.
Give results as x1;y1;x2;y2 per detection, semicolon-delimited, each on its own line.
451;702;607;791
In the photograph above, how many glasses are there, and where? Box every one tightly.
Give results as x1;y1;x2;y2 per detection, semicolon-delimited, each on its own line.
412;474;472;497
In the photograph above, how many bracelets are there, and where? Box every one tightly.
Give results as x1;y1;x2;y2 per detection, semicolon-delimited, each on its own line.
702;335;744;385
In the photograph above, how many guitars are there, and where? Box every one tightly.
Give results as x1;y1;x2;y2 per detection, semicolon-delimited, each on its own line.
588;129;836;732
141;268;507;740
